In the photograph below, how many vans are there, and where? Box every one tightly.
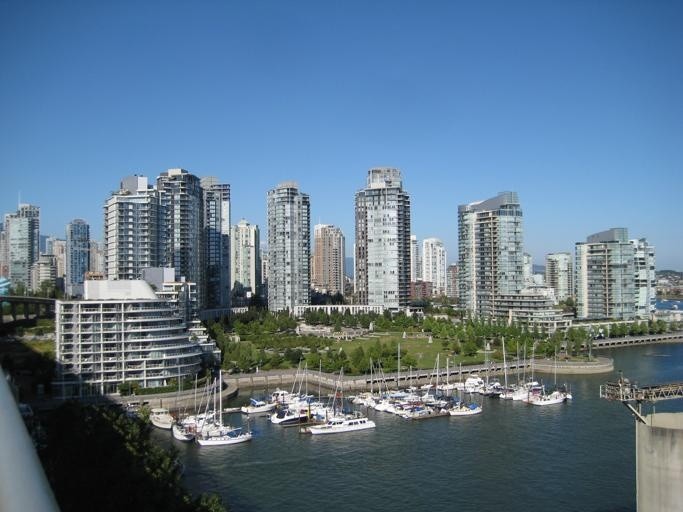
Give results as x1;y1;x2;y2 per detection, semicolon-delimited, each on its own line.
18;404;32;419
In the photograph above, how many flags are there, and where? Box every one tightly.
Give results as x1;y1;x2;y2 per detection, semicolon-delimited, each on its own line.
305;403;311;419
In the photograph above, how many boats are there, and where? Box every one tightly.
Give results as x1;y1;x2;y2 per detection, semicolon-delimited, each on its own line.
150;408;176;429
124;405;144;419
642;351;672;358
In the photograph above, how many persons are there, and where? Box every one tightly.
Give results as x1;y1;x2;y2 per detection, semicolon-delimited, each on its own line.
487;359;496;368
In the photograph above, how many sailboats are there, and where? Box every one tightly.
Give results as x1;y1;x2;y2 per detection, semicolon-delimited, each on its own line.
349;336;573;420
172;367;254;445
240;356;376;435
